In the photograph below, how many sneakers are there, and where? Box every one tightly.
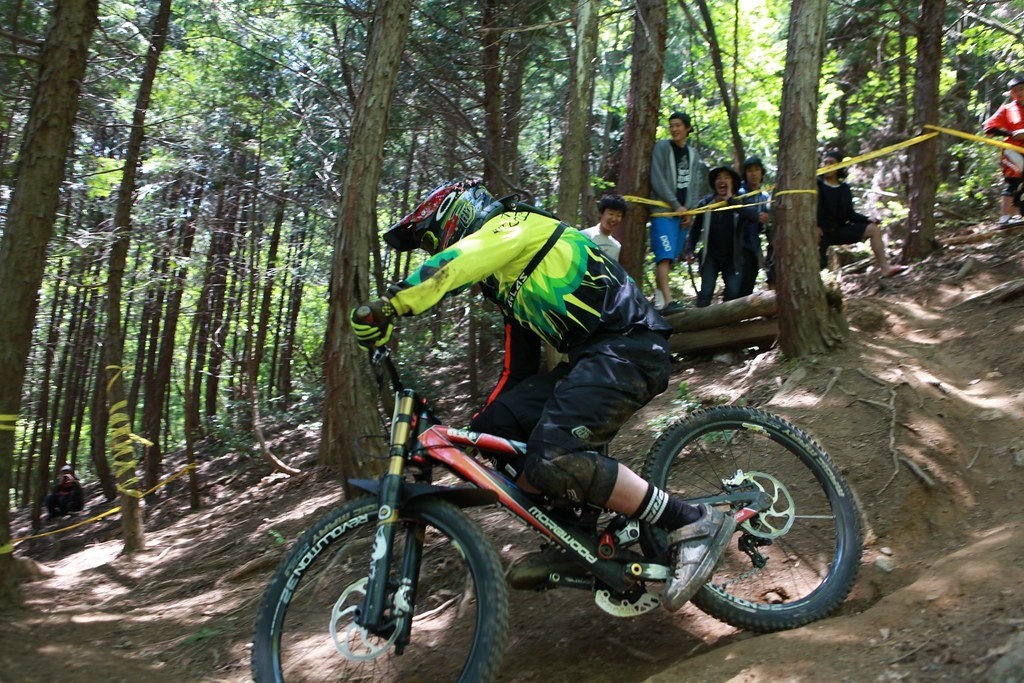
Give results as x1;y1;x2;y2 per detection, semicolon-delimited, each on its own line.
661;502;738;612
652;287;666;311
505;546;585;589
998;215;1023;227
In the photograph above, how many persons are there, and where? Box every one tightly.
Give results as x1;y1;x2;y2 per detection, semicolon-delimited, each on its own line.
349;178;737;610
649;112;698;313
688;156;771;307
983;77;1024;224
578;192;627;267
817;150;909;278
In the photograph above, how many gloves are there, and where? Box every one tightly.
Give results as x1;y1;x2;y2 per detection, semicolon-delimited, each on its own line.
348;293;397;348
448;426;479;455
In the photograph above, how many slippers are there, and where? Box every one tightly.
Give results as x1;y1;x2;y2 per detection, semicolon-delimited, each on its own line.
884;264;909;275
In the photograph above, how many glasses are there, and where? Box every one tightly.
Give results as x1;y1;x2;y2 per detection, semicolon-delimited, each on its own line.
416;231;438;252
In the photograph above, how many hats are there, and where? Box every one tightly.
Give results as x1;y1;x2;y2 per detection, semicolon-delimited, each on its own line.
669;111;694;132
744;156;762;167
709;165;742;195
1007;75;1024;88
824;151;844;162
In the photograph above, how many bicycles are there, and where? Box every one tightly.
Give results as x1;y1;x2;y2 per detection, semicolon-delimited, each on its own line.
250;305;863;683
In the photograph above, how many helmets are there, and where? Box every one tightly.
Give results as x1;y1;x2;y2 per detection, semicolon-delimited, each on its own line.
382;178;519;289
